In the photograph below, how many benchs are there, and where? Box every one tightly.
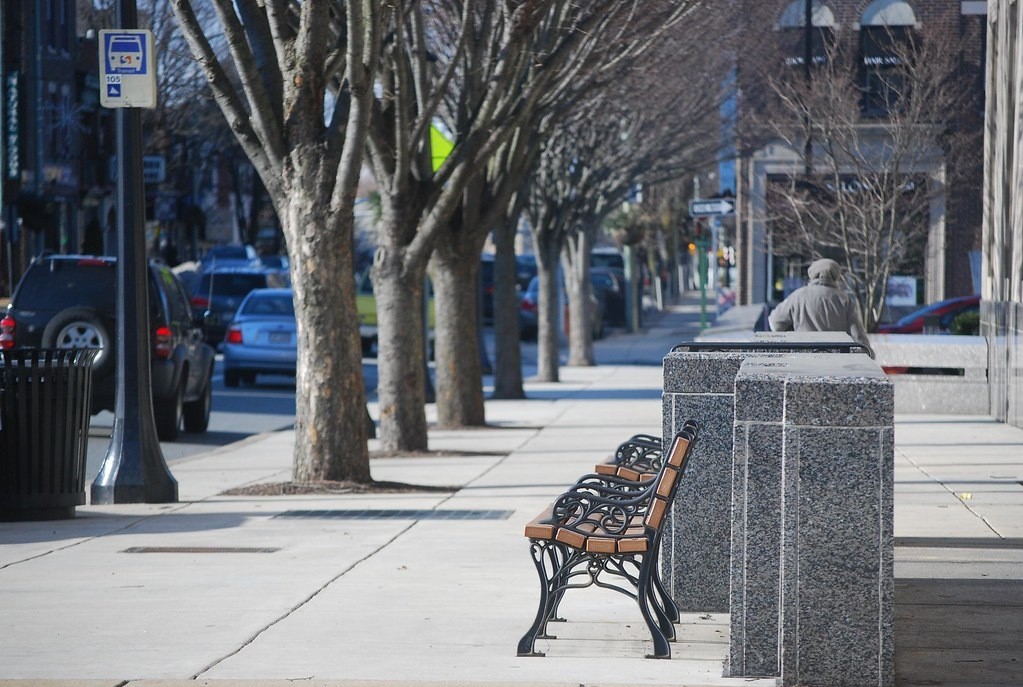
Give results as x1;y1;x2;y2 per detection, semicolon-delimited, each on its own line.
596;434;662;482
517;420;700;660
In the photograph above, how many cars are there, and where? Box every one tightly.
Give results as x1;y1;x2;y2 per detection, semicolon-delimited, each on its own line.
199;242;262;273
482;246;655;322
349;268;438;363
188;269;283;340
521;272;607;342
870;293;983;373
220;289;299;389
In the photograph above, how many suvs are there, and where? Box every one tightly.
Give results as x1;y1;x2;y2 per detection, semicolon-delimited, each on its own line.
0;249;216;441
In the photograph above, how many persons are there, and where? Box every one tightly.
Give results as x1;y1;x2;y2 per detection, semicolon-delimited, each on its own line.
767;258;876;361
